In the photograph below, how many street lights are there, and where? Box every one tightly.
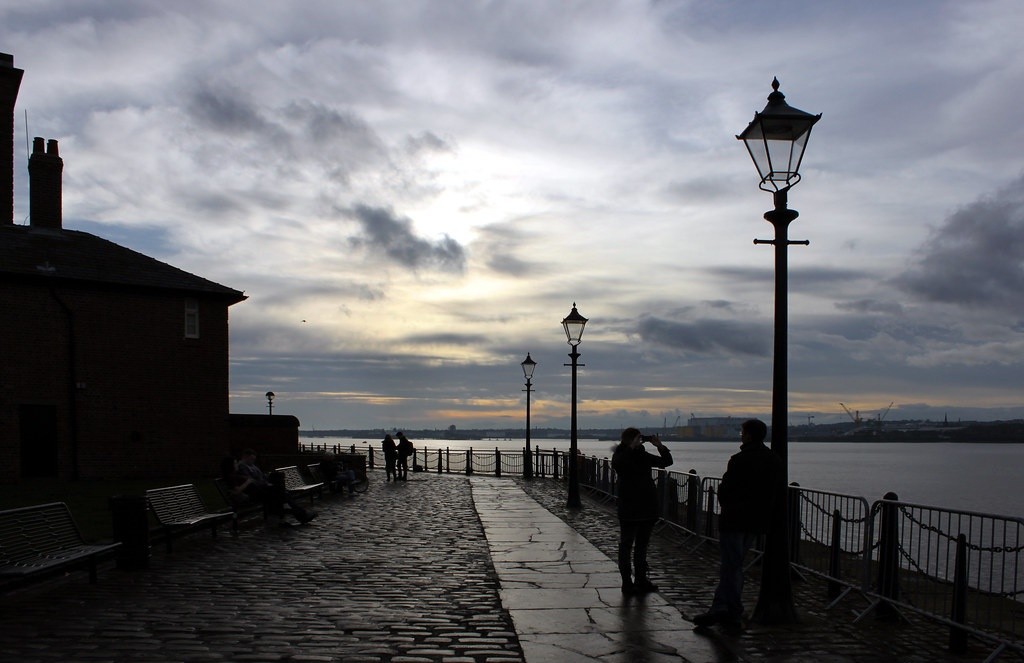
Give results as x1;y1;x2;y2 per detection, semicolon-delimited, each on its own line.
559;301;590;511
735;72;824;625
265;391;276;414
520;351;538;479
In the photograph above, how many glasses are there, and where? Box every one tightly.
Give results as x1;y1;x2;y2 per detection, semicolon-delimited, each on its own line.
739;432;744;436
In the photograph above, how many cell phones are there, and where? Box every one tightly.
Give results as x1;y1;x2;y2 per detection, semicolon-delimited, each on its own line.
642;436;653;441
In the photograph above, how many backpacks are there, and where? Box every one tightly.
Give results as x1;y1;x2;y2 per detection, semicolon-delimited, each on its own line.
405;440;413;456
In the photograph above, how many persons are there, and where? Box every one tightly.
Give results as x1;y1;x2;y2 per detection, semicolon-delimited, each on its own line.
693;419;783;626
611;427;674;593
319;452;339;491
382;434;397;481
395;431;408;481
221;448;313;525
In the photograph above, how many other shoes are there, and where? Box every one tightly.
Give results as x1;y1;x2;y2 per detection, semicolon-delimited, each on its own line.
300;512;318;525
622;581;658;596
693;605;744;627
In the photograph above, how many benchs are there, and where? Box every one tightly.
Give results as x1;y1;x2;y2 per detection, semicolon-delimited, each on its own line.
0;501;123;577
214;474;265;532
307;461;336;500
146;481;238;540
273;465;325;508
